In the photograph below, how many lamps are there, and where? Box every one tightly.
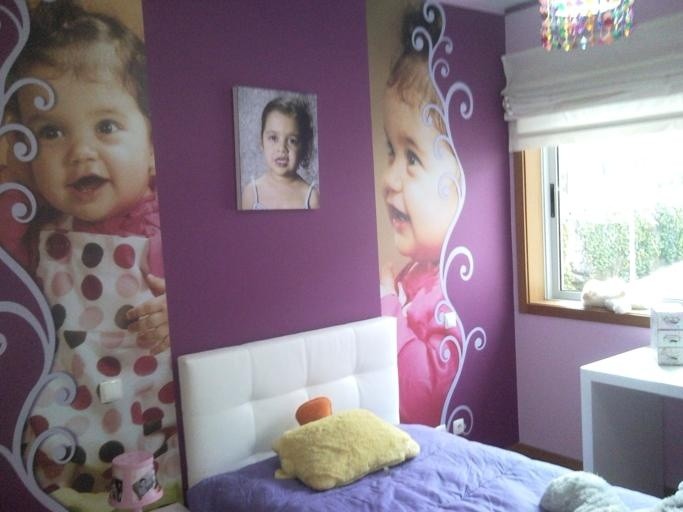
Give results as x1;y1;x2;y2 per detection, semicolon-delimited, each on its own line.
106;451;163;511
537;0;634;53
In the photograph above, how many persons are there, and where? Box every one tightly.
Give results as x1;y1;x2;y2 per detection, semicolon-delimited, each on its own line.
0;0;184;512
241;96;318;210
379;45;463;428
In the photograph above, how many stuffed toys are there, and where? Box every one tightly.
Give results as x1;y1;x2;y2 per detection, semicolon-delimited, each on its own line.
541;470;683;512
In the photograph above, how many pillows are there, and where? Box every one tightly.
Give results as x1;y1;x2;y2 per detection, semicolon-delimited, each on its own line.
271;407;420;490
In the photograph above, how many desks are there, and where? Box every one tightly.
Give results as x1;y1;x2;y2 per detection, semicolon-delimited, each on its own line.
578;345;683;498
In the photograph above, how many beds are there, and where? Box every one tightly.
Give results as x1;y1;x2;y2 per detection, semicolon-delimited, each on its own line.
176;313;663;512
